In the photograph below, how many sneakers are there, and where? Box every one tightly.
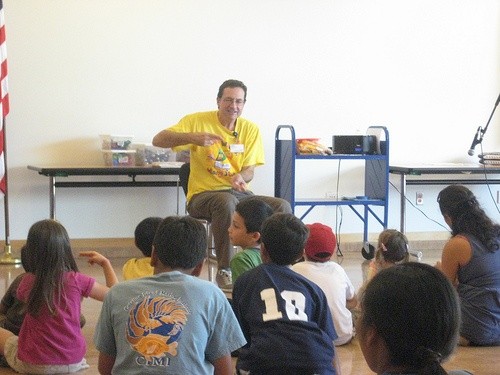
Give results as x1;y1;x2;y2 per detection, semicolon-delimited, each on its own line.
215;267;233;292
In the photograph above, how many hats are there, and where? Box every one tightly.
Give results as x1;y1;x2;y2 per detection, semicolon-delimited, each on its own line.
303;223;336;262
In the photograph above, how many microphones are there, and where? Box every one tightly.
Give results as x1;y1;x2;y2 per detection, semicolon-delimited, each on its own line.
233;132;238;137
468;126;481;156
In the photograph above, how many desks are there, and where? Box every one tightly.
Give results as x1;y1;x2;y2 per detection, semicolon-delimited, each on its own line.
27;164;181;221
389;165;500;235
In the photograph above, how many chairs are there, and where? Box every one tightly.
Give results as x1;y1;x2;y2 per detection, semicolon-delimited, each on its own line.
179;162;219;282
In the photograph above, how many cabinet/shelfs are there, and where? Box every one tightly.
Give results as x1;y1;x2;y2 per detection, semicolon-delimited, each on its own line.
274;125;390;261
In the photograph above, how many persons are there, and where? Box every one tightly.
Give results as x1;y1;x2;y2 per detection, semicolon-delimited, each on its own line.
291;223;357;347
232;213;339;375
122;217;164;281
227;198;273;305
434;185;500;347
367;229;408;283
152;80;292;293
93;214;247;375
355;262;473;375
0;220;119;375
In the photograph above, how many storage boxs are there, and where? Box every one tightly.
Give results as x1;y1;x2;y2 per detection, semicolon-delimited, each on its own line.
99;134;137;166
